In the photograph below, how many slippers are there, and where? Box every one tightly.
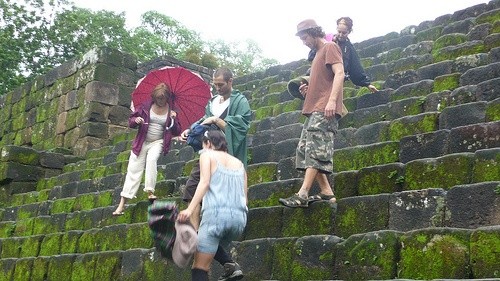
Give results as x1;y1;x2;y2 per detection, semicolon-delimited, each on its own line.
148;195;156;199
113;210;124;215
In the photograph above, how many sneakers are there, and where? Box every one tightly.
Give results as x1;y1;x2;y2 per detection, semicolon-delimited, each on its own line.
218;262;244;281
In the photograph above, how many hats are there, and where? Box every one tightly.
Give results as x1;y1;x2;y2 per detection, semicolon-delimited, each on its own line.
296;19;319;35
172;218;199;269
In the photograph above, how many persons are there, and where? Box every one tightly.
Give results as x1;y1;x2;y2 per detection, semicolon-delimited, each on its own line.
112;82;182;215
306;17;378;93
279;19;344;208
181;67;251;232
178;130;249;281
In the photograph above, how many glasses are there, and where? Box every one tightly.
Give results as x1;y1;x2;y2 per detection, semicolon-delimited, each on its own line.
203;130;211;140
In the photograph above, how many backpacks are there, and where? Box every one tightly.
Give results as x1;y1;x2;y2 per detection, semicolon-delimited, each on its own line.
148;201;177;259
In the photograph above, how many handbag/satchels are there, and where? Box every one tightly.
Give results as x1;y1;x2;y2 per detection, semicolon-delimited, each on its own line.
186;125;211;152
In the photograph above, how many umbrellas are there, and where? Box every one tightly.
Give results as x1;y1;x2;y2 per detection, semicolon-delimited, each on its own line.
129;65;213;136
147;200;178;260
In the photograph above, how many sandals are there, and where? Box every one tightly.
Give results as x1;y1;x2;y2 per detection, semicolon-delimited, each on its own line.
279;193;308;208
308;193;336;204
